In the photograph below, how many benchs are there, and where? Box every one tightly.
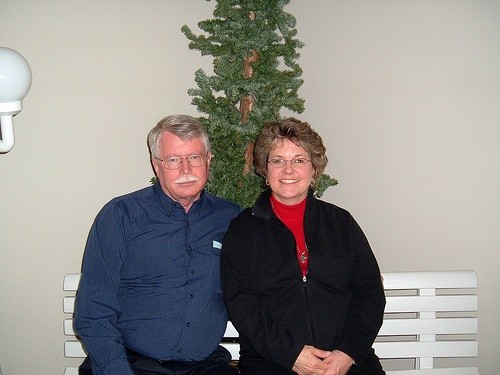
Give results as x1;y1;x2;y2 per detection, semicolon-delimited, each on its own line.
62;270;480;375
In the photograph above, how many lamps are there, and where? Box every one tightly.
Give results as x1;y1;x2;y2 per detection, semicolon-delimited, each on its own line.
0;46;32;153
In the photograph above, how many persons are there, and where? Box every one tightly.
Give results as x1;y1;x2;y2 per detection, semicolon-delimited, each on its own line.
220;117;386;375
75;116;246;375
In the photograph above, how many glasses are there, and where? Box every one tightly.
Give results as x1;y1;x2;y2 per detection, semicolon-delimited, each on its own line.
156;154;208;170
267;158;313;168
